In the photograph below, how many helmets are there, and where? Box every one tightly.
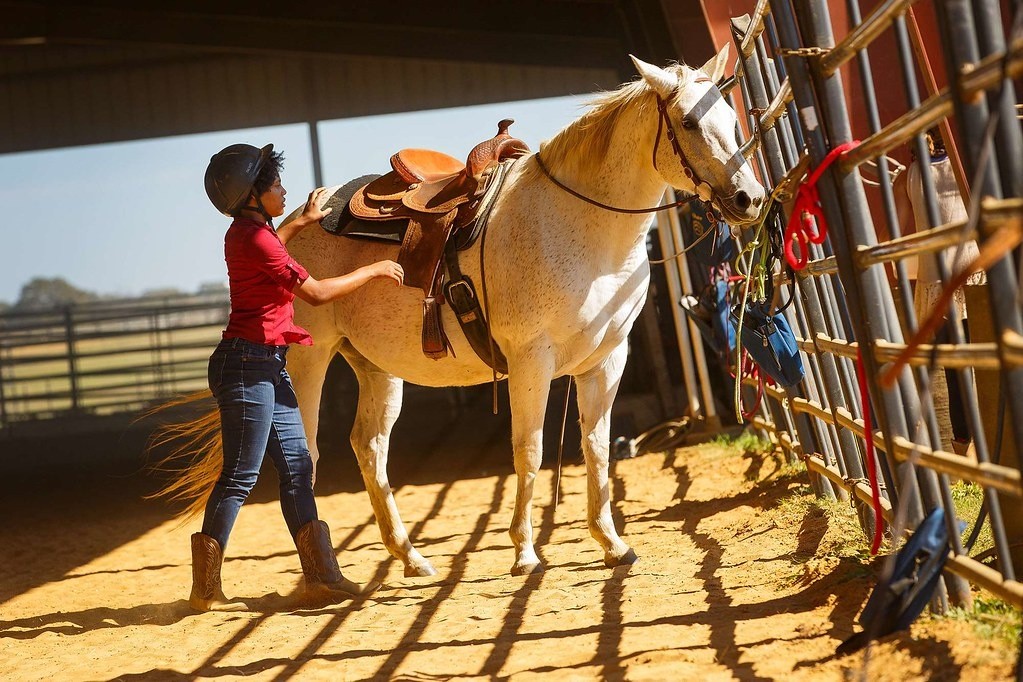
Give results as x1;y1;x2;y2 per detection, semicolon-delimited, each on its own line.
204;143;274;219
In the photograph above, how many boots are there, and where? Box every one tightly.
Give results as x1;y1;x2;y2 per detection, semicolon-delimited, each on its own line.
950;439;972;487
296;519;380;604
189;533;250;612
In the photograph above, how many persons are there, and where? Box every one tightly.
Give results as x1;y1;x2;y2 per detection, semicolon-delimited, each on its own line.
189;143;405;611
907;122;986;488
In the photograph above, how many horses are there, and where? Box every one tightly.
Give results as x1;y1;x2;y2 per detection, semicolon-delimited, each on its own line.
129;41;766;577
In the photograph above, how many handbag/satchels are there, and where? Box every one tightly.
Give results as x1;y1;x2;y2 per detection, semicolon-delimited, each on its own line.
679;281;738;359
860;509;967;632
728;297;805;389
